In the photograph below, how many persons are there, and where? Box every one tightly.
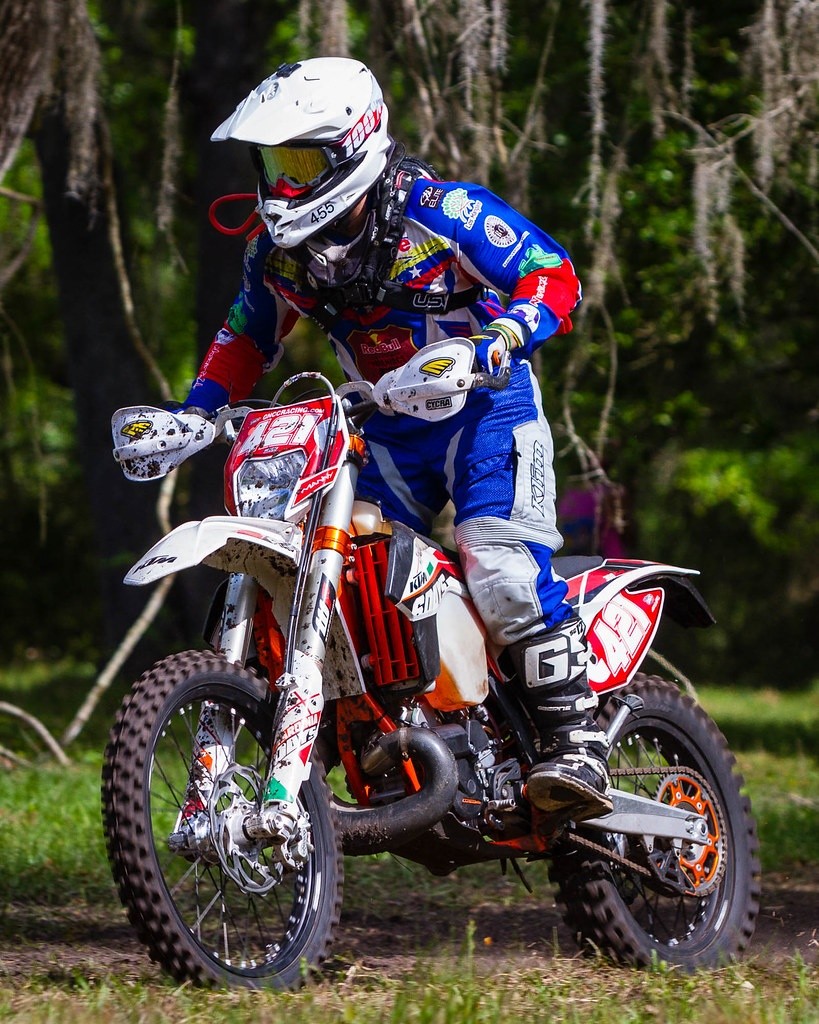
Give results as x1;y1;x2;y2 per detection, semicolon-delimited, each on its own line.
166;55;615;822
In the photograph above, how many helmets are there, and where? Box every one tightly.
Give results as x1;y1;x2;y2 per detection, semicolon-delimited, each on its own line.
209;57;392;249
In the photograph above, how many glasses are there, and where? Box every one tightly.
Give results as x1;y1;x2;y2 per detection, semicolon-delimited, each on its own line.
253;140;337;197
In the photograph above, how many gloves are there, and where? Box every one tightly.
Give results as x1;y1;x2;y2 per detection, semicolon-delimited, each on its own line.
464;326;510;377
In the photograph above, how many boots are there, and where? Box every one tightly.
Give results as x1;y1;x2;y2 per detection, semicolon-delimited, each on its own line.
500;616;613;823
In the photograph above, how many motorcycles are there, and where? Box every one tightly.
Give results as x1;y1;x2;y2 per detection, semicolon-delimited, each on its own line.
103;343;760;994
